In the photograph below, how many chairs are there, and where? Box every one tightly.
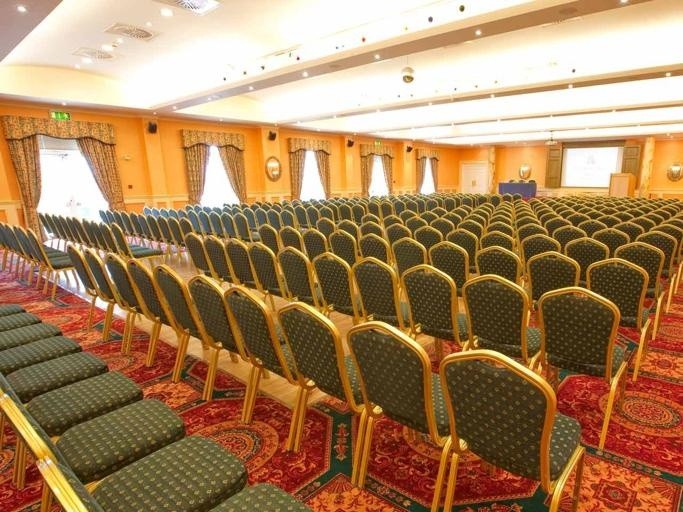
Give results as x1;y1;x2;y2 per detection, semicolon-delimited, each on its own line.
0;178;682;512
